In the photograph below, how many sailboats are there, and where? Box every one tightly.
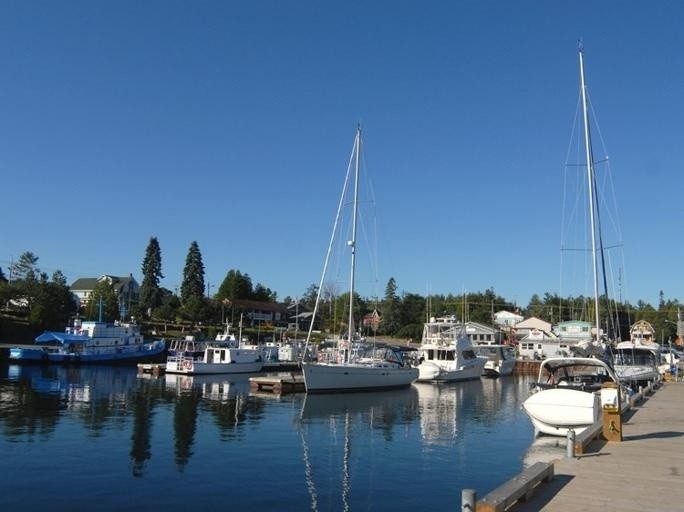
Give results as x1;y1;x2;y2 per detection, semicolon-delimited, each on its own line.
249;120;427;391
294;383;423;512
524;30;635;442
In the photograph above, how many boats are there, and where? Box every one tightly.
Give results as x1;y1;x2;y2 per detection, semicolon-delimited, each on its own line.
611;333;663;384
164;372;254;435
6;290;166;367
5;360;160;405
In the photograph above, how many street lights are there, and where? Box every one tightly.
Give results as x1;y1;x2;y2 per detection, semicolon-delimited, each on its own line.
664;319;677;326
